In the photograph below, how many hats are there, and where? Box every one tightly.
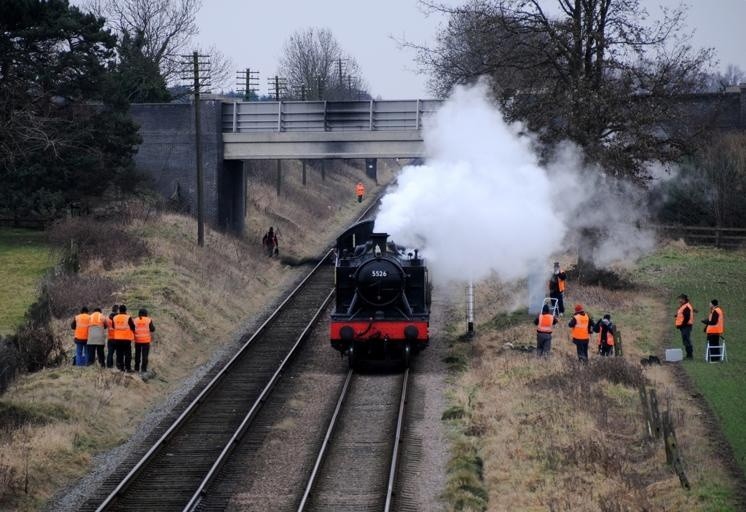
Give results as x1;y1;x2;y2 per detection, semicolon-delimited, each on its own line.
575;304;583;312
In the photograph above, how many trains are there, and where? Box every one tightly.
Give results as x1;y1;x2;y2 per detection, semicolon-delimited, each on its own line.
329;216;433;375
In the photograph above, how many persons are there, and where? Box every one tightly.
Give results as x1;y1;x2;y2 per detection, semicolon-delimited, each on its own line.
701;299;723;362
71;307;90;366
674;293;694;360
594;315;614;356
133;309;155;372
356;182;365;203
263;227;278;258
569;305;594;362
546;261;568;316
87;308;107;369
112;305;135;373
534;305;558;356
106;305;121;369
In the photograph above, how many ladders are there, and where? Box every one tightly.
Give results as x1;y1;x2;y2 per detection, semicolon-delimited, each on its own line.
706;336;729;366
541;298;559;322
